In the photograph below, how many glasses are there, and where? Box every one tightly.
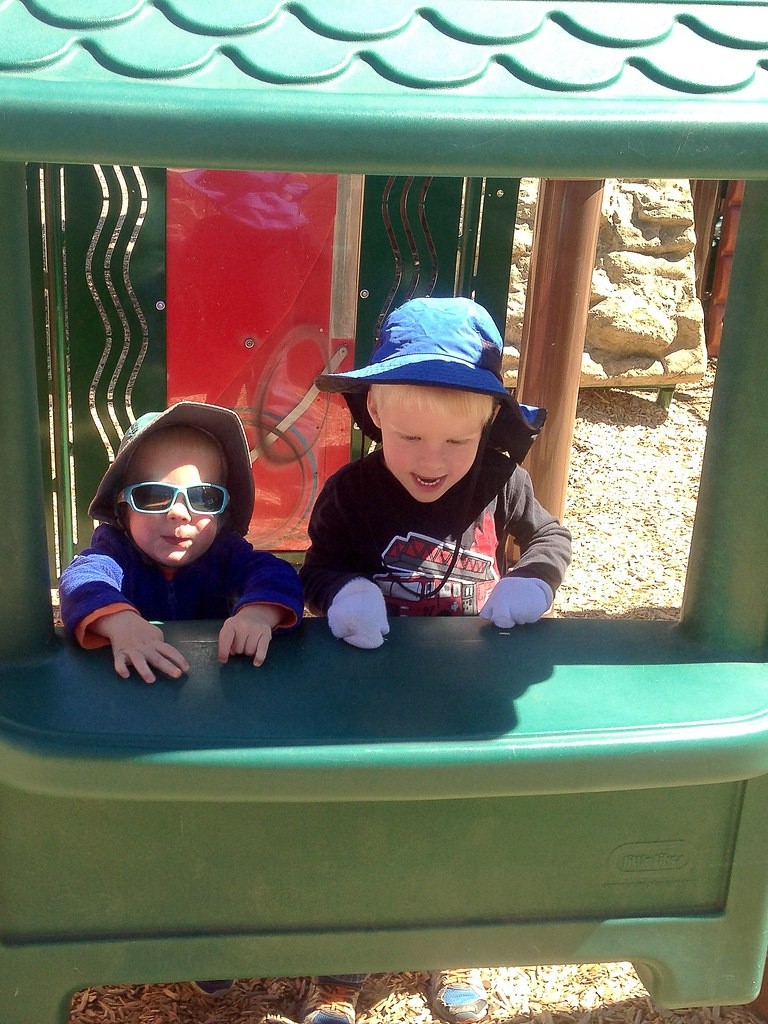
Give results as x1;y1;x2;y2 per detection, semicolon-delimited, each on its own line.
116;481;231;515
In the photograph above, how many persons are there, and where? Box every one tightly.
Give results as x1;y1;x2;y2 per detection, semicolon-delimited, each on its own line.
297;298;572;1024
59;401;301;998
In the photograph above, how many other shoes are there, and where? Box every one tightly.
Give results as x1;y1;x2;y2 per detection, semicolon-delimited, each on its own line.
192;977;235;996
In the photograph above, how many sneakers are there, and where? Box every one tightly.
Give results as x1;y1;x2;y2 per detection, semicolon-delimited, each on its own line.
300;982;363;1024
429;963;488;1024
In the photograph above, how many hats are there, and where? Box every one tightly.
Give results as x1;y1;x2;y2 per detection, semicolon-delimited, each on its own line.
313;296;547;451
88;401;255;537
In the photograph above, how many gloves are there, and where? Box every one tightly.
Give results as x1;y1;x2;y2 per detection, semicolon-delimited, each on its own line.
327;576;389;649
480;575;554;628
